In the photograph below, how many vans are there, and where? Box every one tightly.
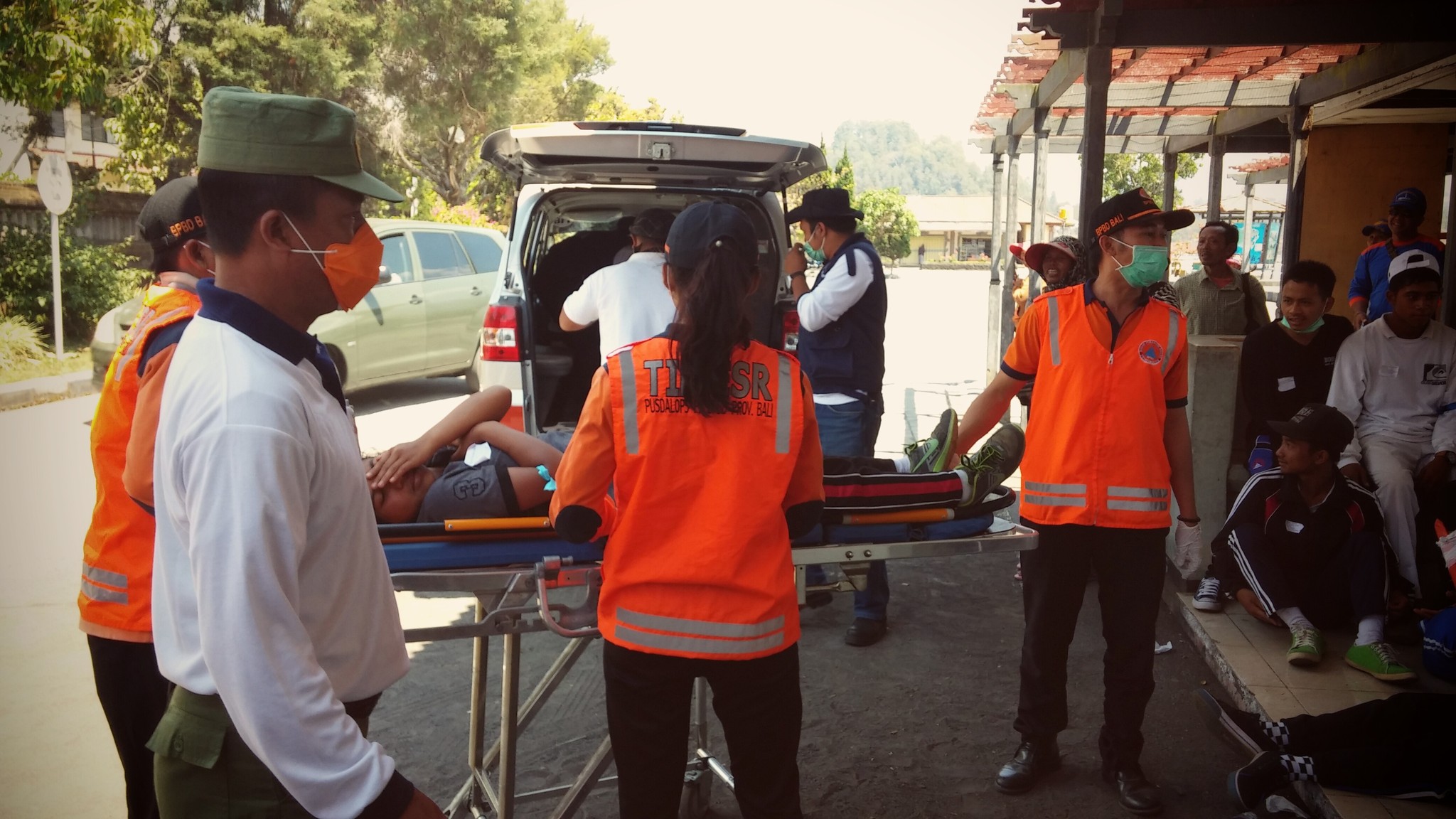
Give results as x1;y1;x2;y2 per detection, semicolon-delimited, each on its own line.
468;119;832;471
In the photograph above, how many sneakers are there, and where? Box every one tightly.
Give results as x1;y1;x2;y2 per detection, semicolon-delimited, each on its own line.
1215;701;1279;755
1286;627;1322;662
1344;641;1418;680
1235;751;1284;810
953;423;1026;509
904;408;959;474
1192;576;1222;610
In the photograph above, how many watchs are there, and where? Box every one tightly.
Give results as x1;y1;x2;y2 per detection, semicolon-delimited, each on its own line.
786;271;806;294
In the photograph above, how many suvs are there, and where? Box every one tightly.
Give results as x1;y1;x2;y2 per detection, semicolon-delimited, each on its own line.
88;220;512;401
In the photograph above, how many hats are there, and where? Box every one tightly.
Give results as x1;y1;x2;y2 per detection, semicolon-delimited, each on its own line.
1387;249;1441;285
1009;242;1031;256
664;200;759;272
1024;235;1084;275
1087;187;1196;251
1362;219;1392;237
1266;402;1354;453
195;85;406;204
784;187;864;225
134;176;207;250
1389;187;1427;217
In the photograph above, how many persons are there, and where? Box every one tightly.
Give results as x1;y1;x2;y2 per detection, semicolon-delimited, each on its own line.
1169;220;1271;336
785;189;891;647
1025;234;1085;296
75;177;225;816
143;86;454;818
524;215;639;432
558;209;680;367
936;189;1211;812
1195;185;1456;819
1190;257;1355;613
544;199;823;818
1009;241;1034;329
362;386;1027;533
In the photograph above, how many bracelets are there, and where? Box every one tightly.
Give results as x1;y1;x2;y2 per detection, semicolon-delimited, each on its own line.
1176;513;1201;523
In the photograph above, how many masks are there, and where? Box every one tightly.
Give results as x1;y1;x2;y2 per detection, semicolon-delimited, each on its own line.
1108;236;1169;288
1280;296;1329;334
279;210;385;313
802;229;827;262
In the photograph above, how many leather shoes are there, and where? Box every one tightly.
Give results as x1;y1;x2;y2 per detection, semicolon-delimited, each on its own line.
1114;761;1163;814
994;742;1060;793
845;616;887;646
